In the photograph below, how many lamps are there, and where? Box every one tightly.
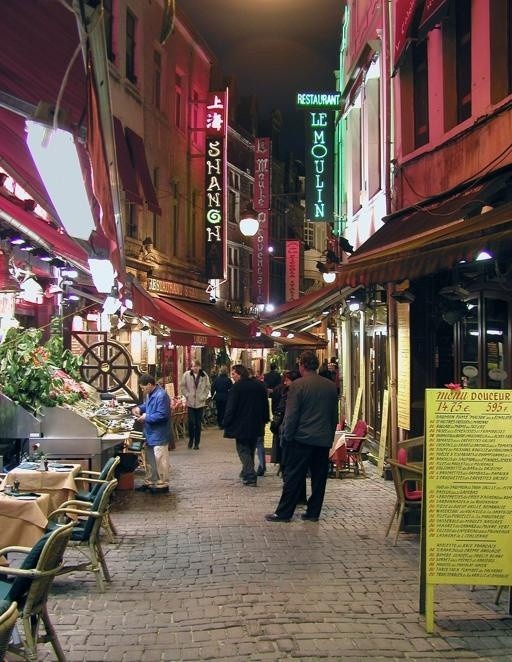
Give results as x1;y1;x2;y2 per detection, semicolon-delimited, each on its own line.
239;207;260;237
299;258;336;283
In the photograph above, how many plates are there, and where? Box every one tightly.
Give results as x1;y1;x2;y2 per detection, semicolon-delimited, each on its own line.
16;496;37;500
55;468;73;472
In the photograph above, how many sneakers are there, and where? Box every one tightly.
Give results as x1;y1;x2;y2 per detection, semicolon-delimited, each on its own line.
135;485;149;492
243;480;258;487
148;487;169;494
264;513;290;522
301;513;319;522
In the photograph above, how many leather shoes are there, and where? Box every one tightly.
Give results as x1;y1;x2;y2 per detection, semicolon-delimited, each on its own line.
188;440;193;448
194;446;199;449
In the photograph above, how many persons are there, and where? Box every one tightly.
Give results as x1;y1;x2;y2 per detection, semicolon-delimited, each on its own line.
271;371;307;507
264;350;337;522
265;363;281;387
180;360;211;449
249;368;270;476
222;365;266;484
132;375;171;493
210;365;233;430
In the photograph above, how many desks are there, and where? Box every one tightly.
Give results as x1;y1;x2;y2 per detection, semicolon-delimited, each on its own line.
0;492;50;584
0;461;81;523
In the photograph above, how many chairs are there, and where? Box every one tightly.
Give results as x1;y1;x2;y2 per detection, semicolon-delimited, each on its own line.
328;419;368;480
41;478;119;595
336;415;347;431
0;519;77;661
72;455;121;545
384;455;423;549
